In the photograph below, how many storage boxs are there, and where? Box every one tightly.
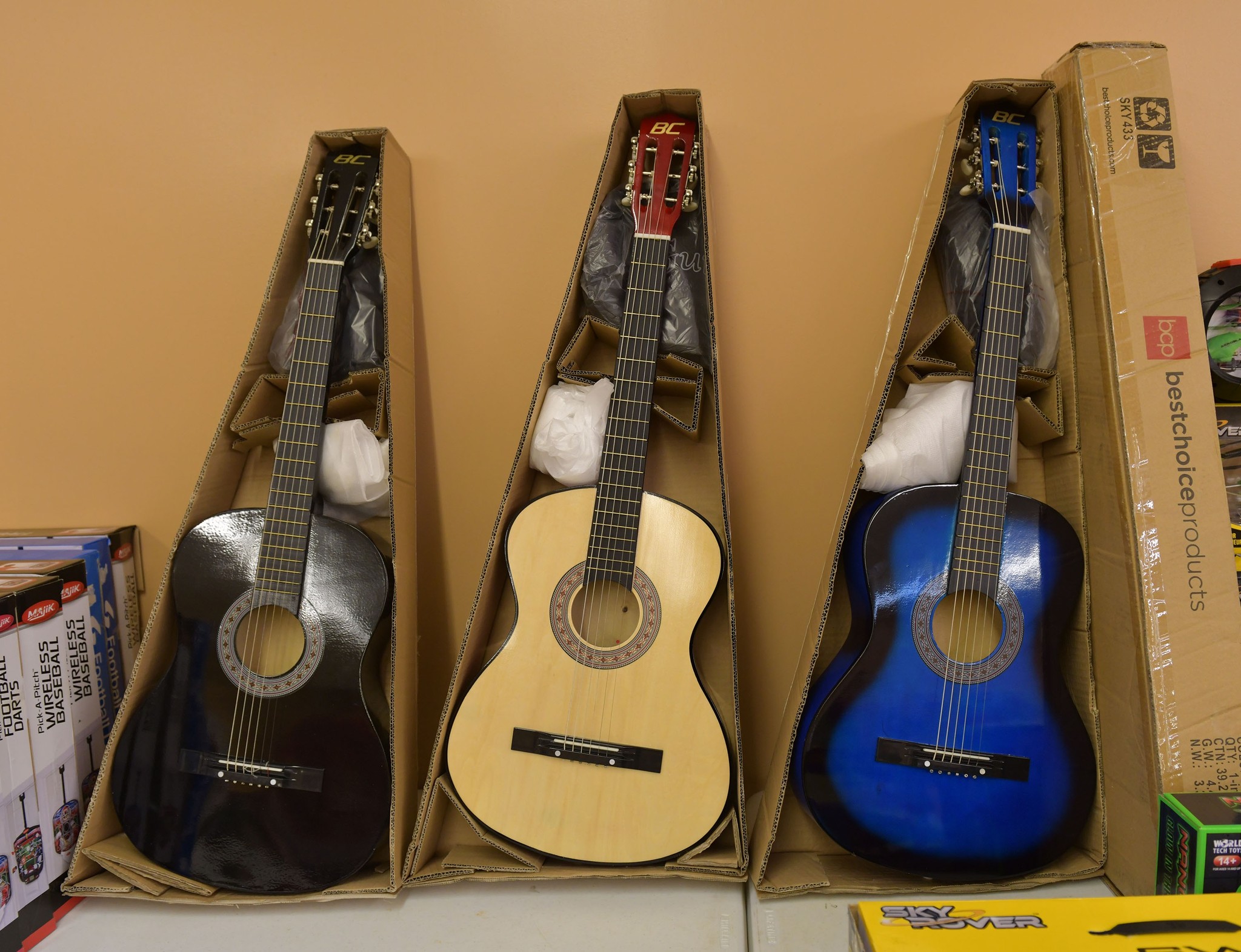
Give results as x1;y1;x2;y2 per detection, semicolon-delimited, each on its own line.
848;889;1241;952
1040;39;1240;896
400;87;751;881
1155;791;1241;895
59;124;418;905
755;80;1111;896
0;528;143;931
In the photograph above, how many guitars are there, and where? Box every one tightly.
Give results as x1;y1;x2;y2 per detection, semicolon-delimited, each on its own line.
790;108;1097;883
445;115;737;868
107;145;393;899
